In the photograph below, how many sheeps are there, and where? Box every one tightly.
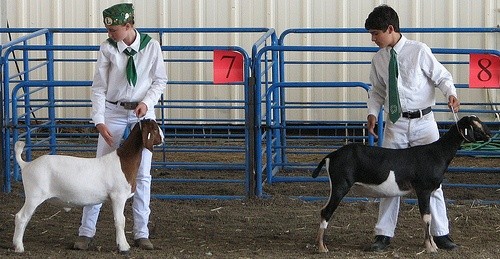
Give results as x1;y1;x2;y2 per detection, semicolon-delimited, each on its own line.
312;116;490;252
12;118;164;252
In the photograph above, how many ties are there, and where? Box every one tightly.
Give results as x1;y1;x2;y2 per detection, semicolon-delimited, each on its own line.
106;33;152;87
388;48;402;125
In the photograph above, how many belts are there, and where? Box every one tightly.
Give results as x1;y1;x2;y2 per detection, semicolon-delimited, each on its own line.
402;107;432;119
105;99;140;110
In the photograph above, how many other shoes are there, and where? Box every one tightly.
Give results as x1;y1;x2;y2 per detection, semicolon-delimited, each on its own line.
361;233;393;253
432;234;458;250
72;235;94;251
134;237;154;251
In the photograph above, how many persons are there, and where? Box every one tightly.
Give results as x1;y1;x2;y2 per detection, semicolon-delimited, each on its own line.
365;4;462;251
72;3;167;251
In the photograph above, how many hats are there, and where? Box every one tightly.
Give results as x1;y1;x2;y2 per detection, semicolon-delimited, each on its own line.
102;3;135;27
